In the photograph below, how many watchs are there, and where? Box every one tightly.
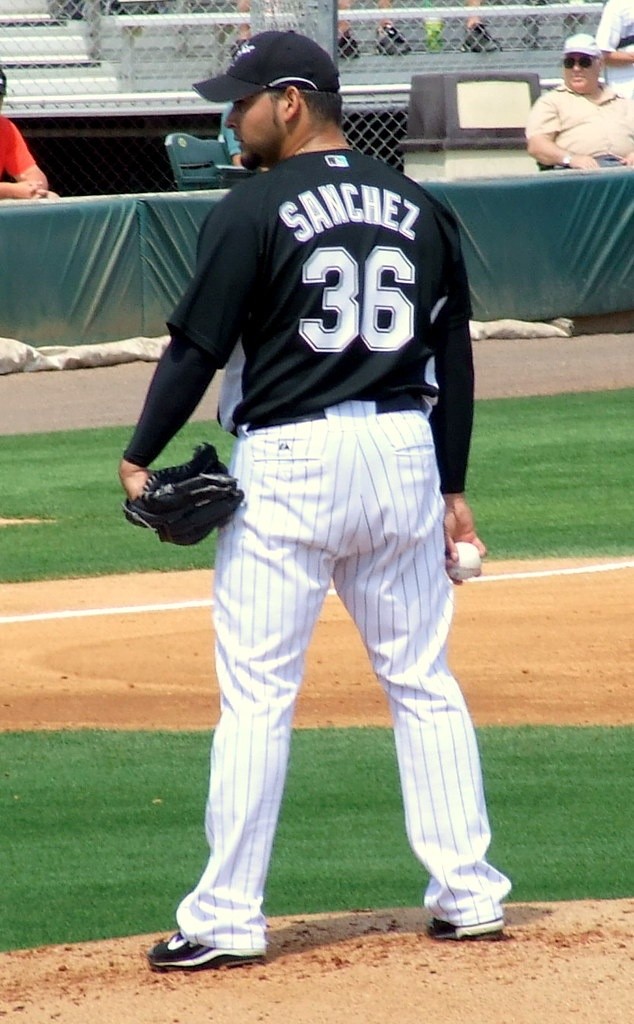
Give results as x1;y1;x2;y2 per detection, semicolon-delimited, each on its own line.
562;151;577;167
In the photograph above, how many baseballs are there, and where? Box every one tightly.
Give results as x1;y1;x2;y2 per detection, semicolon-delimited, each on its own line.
445;539;481;580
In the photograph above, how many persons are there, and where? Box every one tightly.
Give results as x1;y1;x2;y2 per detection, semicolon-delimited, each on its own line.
120;32;510;972
0;68;61;203
218;0;634;173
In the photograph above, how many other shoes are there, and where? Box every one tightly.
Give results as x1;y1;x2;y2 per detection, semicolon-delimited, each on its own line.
375;29;409;54
523;17;542;48
337;38;358;59
460;24;500;51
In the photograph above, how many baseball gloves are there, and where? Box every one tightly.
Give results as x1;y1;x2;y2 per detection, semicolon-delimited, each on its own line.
123;441;245;550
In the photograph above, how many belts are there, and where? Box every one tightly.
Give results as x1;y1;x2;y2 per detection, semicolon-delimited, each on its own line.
247;395;425;430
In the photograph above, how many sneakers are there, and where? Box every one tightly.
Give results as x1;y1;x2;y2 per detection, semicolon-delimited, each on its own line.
147;932;266;972
426;917;505;940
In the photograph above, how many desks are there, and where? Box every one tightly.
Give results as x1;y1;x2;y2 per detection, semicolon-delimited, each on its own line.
0;166;634;374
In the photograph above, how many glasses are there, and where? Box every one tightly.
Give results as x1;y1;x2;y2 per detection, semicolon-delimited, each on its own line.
563;57;592;68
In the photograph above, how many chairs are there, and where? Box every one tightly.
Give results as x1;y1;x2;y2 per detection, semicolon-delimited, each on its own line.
165;133;229;190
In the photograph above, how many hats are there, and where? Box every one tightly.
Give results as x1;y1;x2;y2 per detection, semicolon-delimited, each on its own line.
0;71;7;94
561;33;604;62
192;31;341;103
